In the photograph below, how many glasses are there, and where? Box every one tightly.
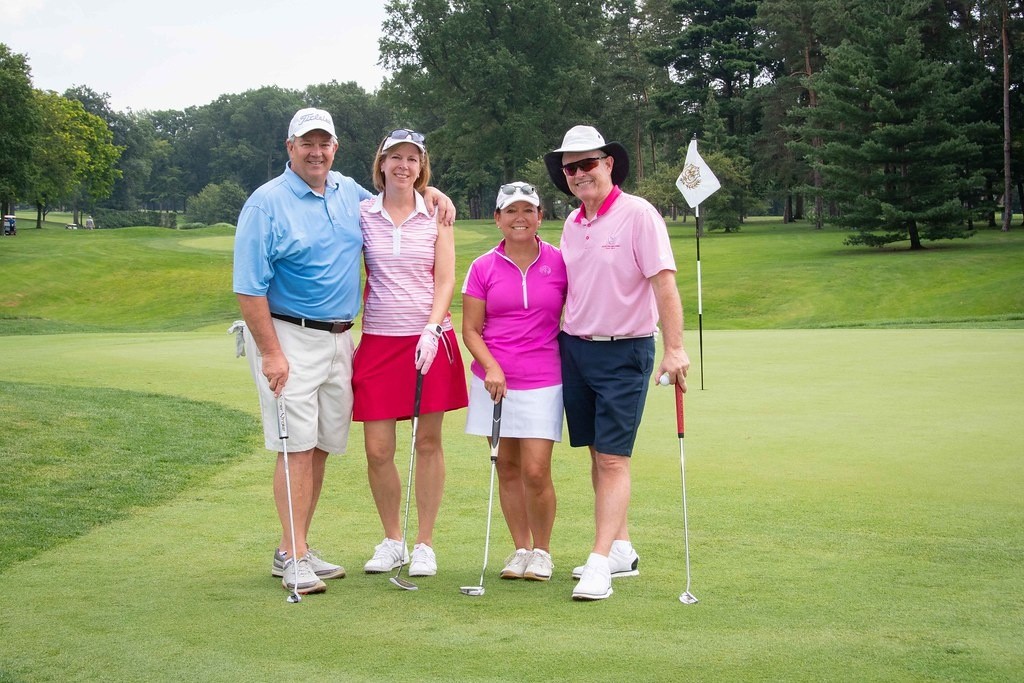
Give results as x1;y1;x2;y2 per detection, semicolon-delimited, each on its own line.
502;184;535;195
381;129;427;154
560;155;608;176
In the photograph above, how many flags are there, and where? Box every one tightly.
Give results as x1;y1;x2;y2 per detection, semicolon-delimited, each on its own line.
675;142;721;208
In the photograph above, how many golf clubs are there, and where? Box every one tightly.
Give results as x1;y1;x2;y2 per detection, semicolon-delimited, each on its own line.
275;391;302;603
675;378;699;605
388;368;423;592
458;394;504;597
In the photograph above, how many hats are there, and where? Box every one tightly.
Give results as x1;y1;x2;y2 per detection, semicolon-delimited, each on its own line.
288;108;337;139
544;126;630;196
381;129;426;153
496;181;539;209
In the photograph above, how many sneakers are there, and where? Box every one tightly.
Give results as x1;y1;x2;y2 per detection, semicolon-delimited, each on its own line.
572;547;640;579
571;561;613;599
409;543;437;576
272;542;345;579
364;537;409;573
500;549;531;580
281;557;326;594
523;550;554;581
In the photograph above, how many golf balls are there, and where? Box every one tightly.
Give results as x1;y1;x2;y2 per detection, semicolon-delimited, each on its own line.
660;374;670;386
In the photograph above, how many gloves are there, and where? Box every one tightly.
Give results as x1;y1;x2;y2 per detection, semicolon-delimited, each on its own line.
415;329;438;376
228;320;247;357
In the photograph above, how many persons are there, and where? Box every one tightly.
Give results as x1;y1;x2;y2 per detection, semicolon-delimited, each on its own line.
460;181;569;580
358;129;469;577
5;217;16;236
232;107;456;595
481;125;692;602
86;216;95;229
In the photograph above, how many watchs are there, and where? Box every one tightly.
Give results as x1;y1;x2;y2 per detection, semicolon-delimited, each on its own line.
425;324;443;338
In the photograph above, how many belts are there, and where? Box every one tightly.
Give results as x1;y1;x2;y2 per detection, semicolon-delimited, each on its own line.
269;312;355;334
580;333;653;342
